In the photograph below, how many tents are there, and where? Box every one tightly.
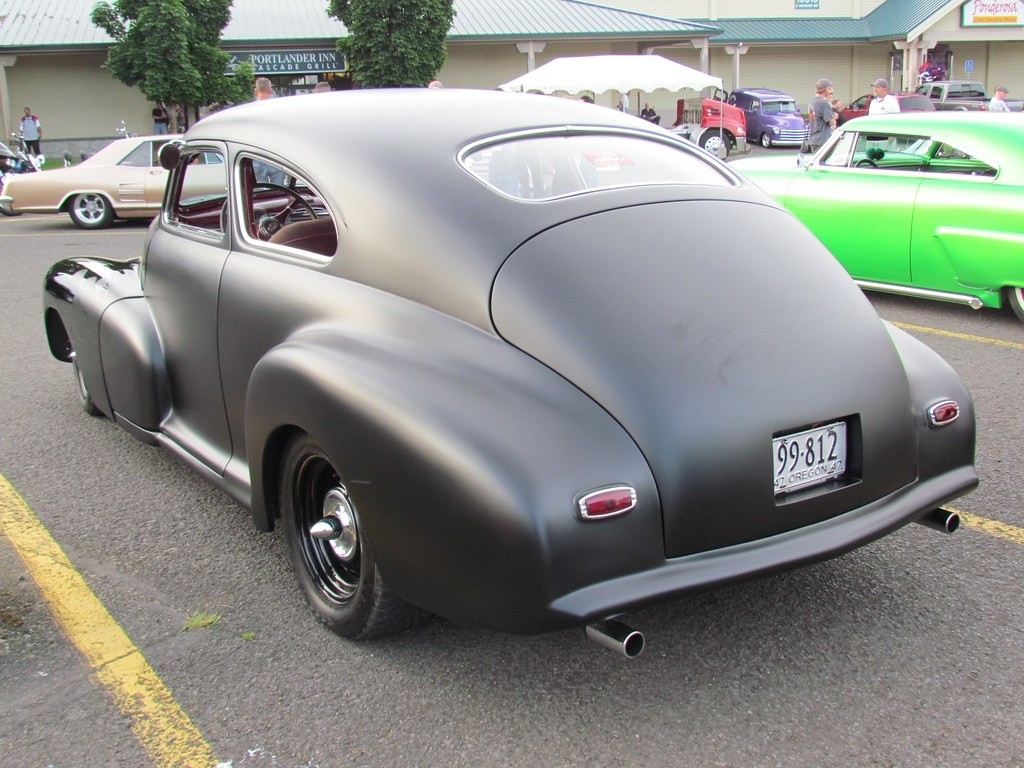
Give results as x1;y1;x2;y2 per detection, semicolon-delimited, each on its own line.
498;54;723;138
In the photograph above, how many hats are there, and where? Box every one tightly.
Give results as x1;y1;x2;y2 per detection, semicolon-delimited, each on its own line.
870;79;888;88
815;79;834;87
997;86;1009;93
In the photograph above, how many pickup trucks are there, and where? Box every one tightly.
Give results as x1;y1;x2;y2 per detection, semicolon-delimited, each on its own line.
913;81;1024;110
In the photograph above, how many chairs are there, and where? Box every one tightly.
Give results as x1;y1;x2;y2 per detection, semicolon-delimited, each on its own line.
267;218;338;257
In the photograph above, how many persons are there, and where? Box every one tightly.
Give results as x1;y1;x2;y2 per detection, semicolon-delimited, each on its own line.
252;78;284;187
618;91;631;114
641;102;661;125
152;102;185;134
428;81;443;87
866;79;901;152
315;81;331;93
580;95;594;104
989;85;1011;112
20;107;42;157
808;78;842;154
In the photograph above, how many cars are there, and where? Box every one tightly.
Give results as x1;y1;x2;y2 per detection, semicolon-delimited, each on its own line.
725;109;1024;326
834;92;937;128
44;86;978;658
0;135;229;230
729;87;811;149
